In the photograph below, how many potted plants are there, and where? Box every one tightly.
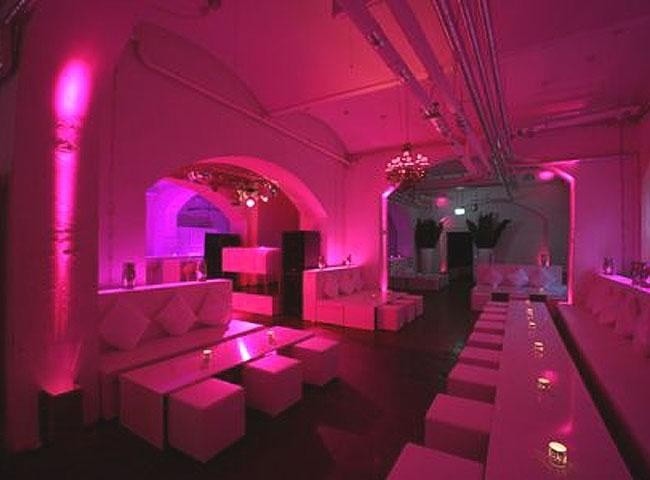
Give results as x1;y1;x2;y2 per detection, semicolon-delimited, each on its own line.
462;211;516;285
413;214;443;274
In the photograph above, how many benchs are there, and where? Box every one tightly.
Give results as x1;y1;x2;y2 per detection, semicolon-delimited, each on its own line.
302;262;364;329
96;275;263;378
556;272;650;454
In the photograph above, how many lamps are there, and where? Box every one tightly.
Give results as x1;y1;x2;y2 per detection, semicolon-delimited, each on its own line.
194;165;282;212
379;77;430;194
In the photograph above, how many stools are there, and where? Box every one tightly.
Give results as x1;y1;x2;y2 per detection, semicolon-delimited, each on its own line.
171;378;246;465
446;362;497;401
385;441;484;480
376;295;423;333
243;355;304;416
459;301;508;368
423;392;495;455
293;336;342;387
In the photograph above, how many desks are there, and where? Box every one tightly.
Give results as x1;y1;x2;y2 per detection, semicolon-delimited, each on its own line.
346;291;407;333
118;324;314;448
486;300;631;480
